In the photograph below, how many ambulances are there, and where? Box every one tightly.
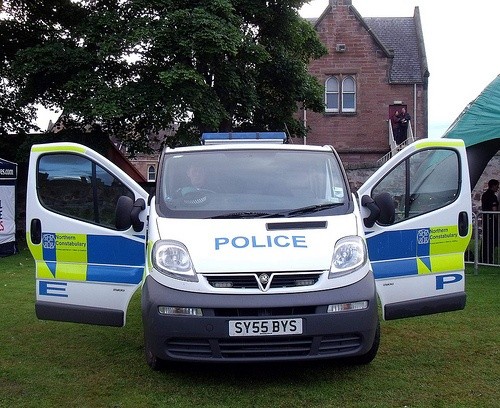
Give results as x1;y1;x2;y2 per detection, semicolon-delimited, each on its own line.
25;132;475;369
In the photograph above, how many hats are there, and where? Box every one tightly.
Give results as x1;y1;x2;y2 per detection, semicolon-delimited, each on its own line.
182;191;210;207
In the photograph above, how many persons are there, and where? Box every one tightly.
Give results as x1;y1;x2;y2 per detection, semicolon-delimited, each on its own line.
478;179;500;267
391;107;411;145
187;164;208;187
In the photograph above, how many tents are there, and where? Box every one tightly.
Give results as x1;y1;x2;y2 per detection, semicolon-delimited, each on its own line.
395;73;500;225
